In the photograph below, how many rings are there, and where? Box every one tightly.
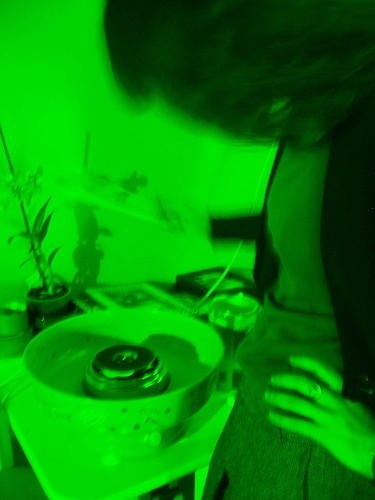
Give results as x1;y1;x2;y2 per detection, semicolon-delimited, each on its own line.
308;381;322;399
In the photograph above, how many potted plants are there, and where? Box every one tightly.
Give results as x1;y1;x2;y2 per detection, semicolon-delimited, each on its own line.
0;127;77;333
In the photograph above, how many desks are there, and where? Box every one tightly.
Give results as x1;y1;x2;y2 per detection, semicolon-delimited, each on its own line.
1;270;262;500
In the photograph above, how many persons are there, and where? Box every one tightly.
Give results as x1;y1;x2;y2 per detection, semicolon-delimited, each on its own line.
102;1;374;500
